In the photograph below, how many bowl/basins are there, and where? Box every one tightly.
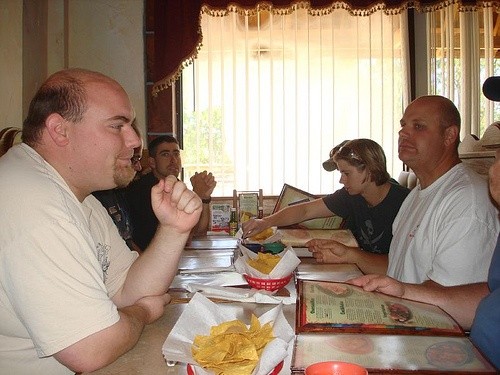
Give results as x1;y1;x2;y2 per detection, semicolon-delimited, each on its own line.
303;361;368;375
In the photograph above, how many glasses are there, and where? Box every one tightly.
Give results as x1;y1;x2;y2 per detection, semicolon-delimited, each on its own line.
132;157;142;164
339;146;363;163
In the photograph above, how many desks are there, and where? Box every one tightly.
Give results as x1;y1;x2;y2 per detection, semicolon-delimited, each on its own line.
74;234;500;375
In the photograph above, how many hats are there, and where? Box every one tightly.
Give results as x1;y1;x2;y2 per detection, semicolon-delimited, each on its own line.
322;140;351;172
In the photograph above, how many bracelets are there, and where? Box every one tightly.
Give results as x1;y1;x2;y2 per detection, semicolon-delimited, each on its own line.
202;197;211;204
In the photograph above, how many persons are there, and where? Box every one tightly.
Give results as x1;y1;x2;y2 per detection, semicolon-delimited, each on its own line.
242;138;411;255
90;124;143;257
345;164;500;370
305;95;500;287
130;135;217;246
0;67;204;375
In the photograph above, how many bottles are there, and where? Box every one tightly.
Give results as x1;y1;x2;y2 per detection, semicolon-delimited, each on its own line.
229;208;238;236
257;207;263;219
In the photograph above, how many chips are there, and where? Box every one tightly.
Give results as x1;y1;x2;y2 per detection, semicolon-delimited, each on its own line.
247;252;282;274
191;313;274;375
248;227;274;241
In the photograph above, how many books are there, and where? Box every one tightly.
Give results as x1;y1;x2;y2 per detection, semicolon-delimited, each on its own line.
290;279;498;375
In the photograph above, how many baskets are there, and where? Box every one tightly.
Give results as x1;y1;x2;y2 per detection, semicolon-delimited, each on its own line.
243;273;293;290
